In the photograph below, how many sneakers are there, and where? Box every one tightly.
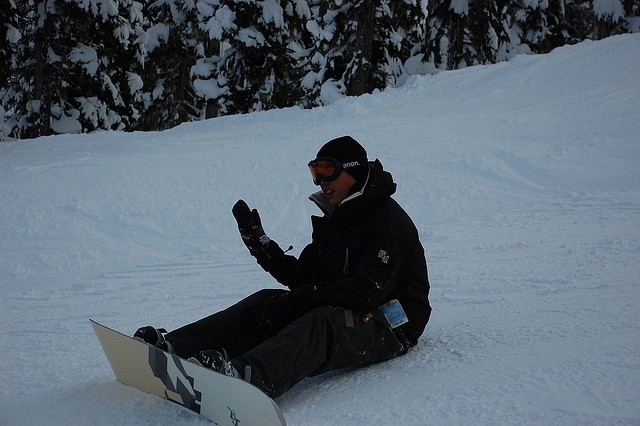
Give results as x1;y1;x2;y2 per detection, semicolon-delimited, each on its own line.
133;326;177;357
199;349;245;379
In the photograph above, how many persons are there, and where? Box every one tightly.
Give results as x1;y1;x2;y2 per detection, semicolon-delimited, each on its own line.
133;136;432;399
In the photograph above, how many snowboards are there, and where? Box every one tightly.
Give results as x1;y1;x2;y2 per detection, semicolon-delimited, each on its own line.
89;319;286;426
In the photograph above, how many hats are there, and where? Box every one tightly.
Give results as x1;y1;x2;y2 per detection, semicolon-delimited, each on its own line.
316;135;368;174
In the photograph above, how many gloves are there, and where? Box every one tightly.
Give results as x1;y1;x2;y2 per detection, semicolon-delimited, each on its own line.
232;199;293;272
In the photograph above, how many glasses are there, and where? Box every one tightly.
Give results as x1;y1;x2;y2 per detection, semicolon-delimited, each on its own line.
307;157;368;186
